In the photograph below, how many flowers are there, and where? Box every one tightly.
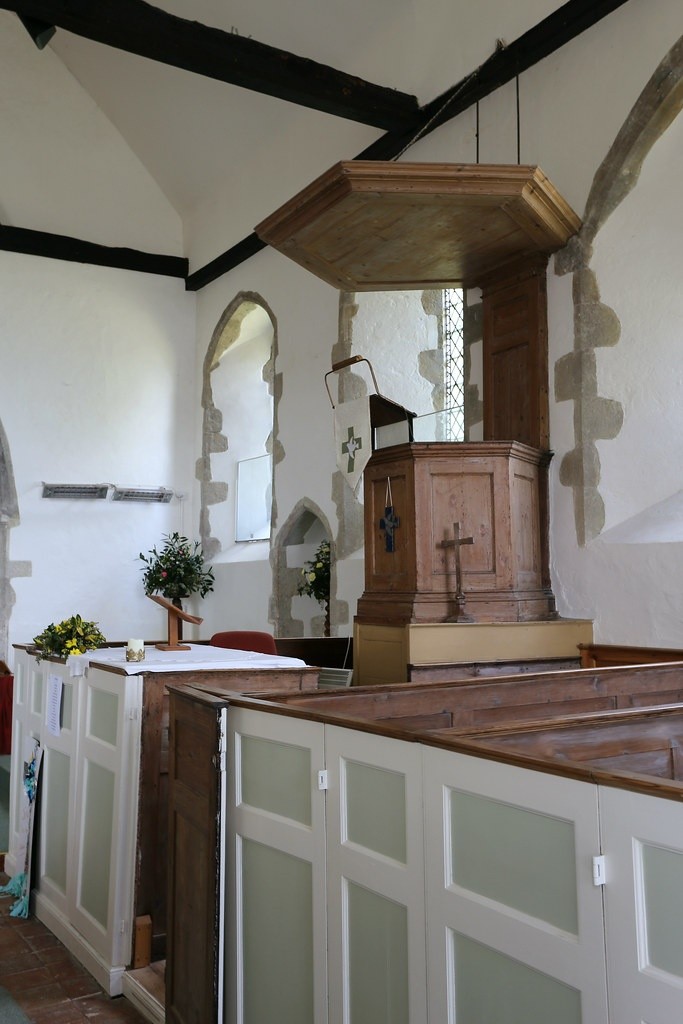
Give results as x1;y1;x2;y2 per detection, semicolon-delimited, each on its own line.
33;614;106;665
298;539;330;604
140;533;216;598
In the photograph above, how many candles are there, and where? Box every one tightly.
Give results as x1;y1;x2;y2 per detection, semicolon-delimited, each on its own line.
126;640;145;662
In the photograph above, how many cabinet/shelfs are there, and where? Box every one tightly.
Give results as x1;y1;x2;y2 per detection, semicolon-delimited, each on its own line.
4;643;320;996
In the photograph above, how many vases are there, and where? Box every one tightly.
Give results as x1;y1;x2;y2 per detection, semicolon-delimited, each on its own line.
324;600;330;637
162;594;190;640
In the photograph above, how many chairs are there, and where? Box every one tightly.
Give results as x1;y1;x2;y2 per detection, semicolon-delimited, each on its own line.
210;631;277;655
